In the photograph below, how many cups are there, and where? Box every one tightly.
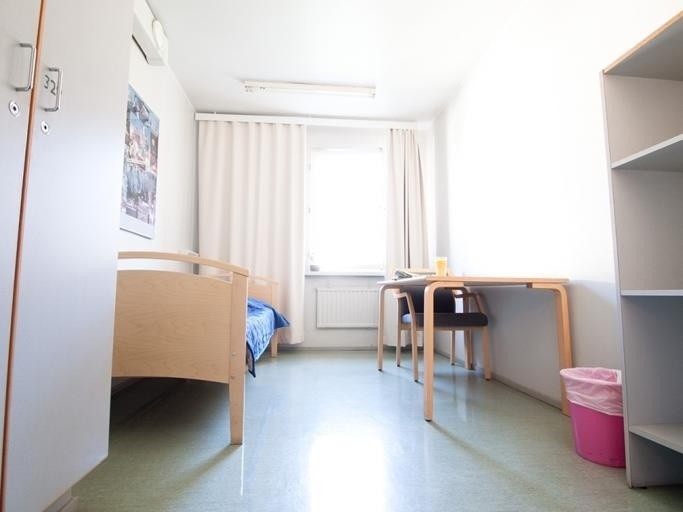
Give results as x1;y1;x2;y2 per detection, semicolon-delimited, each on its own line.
434;257;447;277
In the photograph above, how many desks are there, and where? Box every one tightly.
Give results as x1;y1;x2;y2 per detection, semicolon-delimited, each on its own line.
377;275;575;423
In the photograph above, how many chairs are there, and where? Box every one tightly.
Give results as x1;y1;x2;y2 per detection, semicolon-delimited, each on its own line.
391;266;493;382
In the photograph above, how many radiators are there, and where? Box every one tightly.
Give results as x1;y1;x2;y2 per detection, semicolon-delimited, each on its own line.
316;289;378;329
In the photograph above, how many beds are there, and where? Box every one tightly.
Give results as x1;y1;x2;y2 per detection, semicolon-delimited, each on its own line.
111;250;282;455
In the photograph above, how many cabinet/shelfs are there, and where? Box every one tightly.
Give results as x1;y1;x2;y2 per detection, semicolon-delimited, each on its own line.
598;10;683;489
0;1;136;511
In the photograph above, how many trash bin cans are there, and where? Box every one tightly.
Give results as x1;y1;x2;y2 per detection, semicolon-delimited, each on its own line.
560;367;625;467
